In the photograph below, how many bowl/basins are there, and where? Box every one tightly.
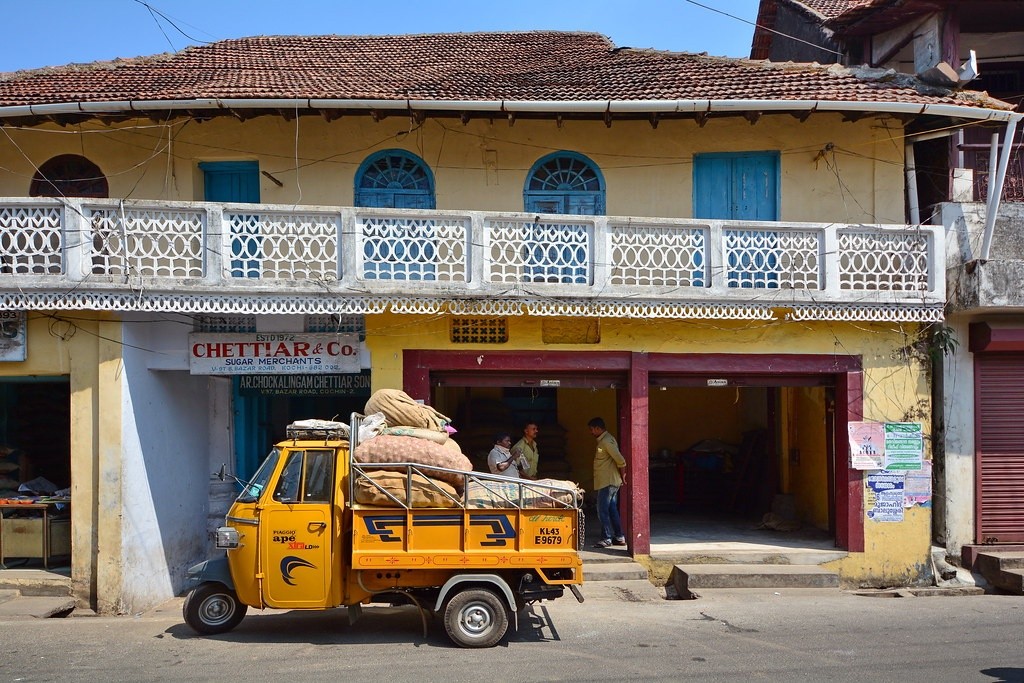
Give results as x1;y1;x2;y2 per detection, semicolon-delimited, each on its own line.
0;498;8;505
7;500;19;504
20;499;33;504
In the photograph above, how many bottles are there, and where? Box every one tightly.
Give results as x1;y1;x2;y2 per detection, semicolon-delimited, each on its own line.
519;453;530;470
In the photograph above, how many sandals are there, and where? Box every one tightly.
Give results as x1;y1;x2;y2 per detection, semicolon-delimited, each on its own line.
594;540;613;548
613;538;626;546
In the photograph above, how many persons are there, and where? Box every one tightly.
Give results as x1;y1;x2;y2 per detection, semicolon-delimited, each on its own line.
588;418;627;548
488;421;539;481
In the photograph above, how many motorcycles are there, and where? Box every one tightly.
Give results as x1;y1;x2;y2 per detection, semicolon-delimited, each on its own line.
182;411;586;649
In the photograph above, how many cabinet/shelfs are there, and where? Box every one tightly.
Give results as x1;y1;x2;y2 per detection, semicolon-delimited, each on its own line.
0;503;71;570
